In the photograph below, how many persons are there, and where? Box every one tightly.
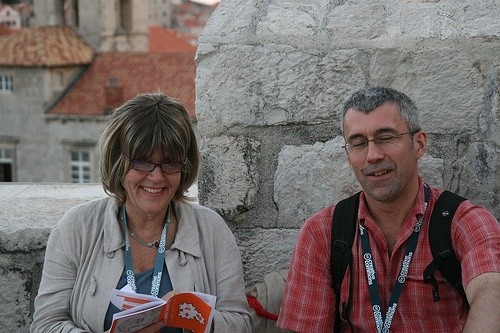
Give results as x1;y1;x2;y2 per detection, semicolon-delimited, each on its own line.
277;86;500;333
30;93;253;333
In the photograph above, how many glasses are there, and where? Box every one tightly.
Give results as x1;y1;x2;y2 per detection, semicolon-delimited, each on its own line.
130;158;188;175
342;131;416;154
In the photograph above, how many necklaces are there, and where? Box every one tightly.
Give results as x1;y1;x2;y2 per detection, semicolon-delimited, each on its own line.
127;228;160;250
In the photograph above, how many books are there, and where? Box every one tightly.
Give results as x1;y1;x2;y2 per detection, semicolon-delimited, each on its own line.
108;286;217;333
